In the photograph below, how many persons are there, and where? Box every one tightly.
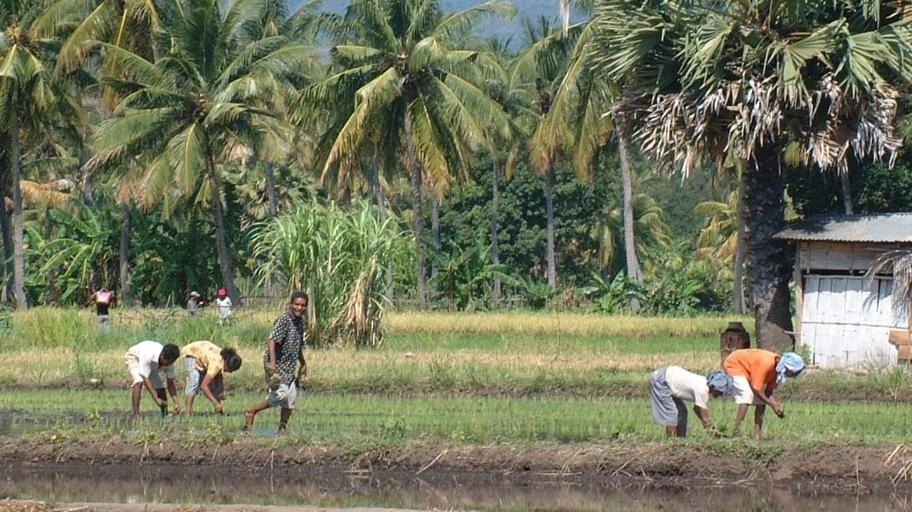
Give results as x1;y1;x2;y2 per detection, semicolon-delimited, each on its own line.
186;291;203;320
723;348;806;440
124;339;181;418
181;340;242;416
216;289;233;326
243;291;308;434
648;364;733;440
93;288;113;330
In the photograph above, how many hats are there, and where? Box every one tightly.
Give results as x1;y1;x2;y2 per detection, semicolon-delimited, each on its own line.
191;291;200;297
218;289;226;296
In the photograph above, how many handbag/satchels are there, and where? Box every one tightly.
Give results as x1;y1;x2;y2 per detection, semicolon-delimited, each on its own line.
94;288;112;305
